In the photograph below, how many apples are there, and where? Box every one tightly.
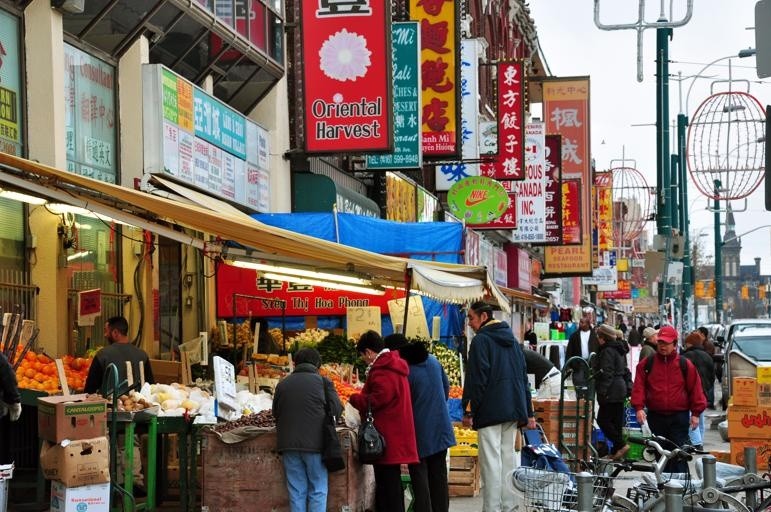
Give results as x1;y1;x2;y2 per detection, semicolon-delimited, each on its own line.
452;427;472;436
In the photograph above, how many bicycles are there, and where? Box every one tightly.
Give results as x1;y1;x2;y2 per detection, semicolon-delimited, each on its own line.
514;434;746;512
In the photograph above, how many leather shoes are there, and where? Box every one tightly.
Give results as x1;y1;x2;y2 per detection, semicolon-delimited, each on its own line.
612;444;630;460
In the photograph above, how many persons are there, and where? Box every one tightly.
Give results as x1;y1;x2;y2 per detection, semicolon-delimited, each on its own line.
522;349;561;399
384;334;458;512
250;316;282;356
84;316;154;497
272;345;345;512
348;329;421;512
460;300;536;512
564;317;717;472
0;350;22;422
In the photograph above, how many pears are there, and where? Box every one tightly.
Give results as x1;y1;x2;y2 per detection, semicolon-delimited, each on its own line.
150;383;209;411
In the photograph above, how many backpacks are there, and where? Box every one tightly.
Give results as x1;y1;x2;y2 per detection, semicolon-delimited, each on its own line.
518;420;575;487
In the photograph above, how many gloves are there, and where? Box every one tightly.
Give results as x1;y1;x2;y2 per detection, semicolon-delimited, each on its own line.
3;402;22;421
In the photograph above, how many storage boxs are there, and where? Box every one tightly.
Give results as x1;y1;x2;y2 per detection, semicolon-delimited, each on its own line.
37;394;107;443
758;384;771;407
733;377;758;406
757;363;771;384
533;400;592;471
730;438;771;470
727;396;771;439
39;436;111;488
50;479;111;512
448;455;480;497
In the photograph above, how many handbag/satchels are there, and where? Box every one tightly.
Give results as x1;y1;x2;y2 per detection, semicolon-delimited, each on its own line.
321;375;346;472
355;394;386;463
624;368;634;397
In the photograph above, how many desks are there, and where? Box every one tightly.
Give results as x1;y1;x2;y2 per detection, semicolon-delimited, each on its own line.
106;416;206;510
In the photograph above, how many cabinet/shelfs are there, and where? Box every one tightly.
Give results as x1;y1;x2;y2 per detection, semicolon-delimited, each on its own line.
199;432;376;512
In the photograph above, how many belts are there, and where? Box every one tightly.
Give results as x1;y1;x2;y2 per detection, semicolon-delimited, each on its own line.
543;371;559;382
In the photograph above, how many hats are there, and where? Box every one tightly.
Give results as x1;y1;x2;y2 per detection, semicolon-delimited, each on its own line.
295;347;322;369
657;325;678;343
643;326;660;337
686;333;701;345
598;323;617;339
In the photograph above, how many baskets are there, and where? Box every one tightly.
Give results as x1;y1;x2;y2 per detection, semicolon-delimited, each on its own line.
519;465;610;512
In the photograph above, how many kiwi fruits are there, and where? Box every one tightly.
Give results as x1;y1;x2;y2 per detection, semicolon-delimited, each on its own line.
105;394;151;412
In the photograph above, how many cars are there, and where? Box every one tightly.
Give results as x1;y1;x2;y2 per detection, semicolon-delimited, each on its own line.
713;319;771;367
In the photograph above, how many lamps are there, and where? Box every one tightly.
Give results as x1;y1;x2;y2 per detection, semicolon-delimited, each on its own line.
223;253;372;286
262;273;386;297
